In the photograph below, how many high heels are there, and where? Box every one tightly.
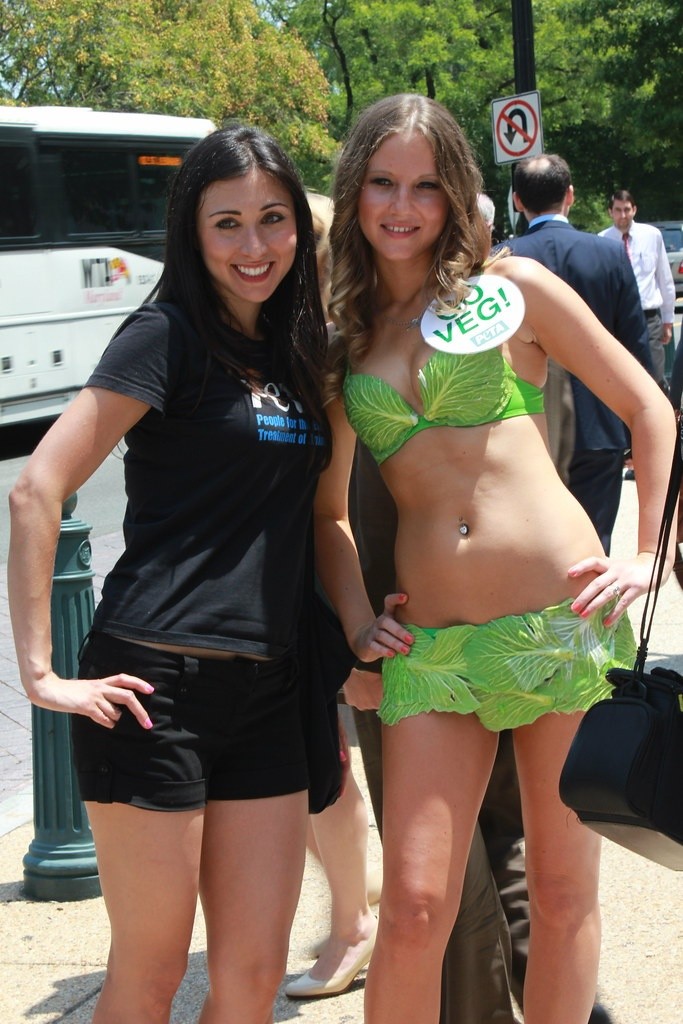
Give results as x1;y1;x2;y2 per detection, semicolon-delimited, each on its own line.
308;875;381;956
285;916;381;997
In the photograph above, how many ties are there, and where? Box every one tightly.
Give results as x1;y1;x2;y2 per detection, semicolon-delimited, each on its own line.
622;233;632;268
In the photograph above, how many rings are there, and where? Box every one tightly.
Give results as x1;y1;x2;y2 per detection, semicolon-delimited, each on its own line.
609;582;621;600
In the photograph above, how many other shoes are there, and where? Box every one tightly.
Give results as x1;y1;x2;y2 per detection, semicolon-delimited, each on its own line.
625;470;636;480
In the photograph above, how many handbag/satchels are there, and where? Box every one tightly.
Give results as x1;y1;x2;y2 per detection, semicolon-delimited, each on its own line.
557;668;683;873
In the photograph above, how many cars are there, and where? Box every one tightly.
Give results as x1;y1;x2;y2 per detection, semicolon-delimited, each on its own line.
645;220;683;300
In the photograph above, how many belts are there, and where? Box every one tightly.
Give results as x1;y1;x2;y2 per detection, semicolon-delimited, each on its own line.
644;309;657;320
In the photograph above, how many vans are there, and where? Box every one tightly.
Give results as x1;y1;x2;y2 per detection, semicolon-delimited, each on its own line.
0;104;216;427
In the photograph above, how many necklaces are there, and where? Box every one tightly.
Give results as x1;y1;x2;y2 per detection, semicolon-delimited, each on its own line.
378;277;438;332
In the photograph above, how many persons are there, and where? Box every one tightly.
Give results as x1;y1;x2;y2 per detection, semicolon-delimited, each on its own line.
4;124;333;1023
284;153;680;1022
302;93;675;1023
